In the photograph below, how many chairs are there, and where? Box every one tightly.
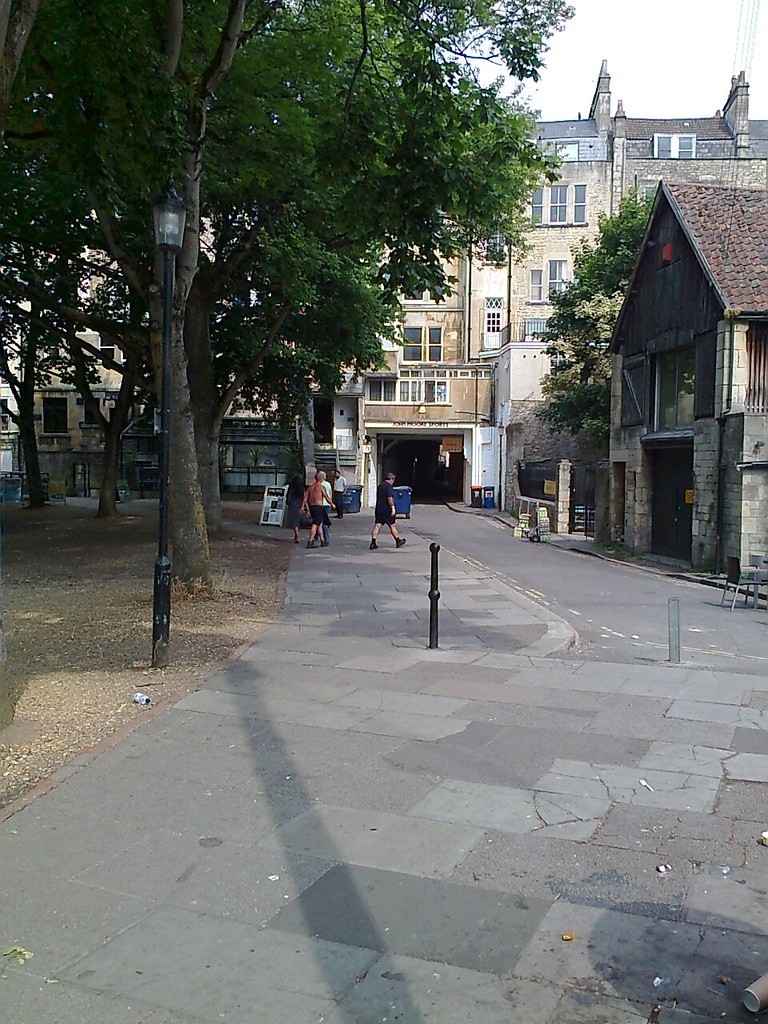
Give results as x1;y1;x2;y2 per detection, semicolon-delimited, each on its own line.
719;555;763;612
745;553;768;605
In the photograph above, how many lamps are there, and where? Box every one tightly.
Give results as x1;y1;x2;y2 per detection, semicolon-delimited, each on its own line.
413;403;427;414
362;434;372;454
755;441;766;449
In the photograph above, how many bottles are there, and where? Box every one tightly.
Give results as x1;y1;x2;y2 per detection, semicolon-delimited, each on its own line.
133;693;151;704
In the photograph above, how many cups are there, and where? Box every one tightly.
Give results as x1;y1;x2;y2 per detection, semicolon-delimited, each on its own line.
742;971;768;1012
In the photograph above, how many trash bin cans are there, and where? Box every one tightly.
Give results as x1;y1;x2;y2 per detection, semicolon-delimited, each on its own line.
392;486;412;513
342;485;363;513
471;486;482;508
482;486;495;508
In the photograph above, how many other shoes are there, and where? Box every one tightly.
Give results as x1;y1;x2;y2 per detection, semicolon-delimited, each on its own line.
396;538;407;548
333;513;343;519
370;544;379;550
295;538;300;543
306;541;319;549
321;539;327;547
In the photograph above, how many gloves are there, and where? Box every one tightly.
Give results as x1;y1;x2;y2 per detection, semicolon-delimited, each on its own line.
391;505;395;516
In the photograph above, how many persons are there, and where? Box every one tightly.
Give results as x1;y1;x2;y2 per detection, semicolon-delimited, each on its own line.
286;476;310;544
370;473;407;550
333;471;347;519
299;473;336;548
314;471;332;545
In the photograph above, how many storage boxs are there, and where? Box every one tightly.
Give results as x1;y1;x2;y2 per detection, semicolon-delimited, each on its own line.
512;515;531;538
539;507;552;542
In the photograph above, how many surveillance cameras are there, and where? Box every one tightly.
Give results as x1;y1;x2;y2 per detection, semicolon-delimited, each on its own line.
757;441;765;446
